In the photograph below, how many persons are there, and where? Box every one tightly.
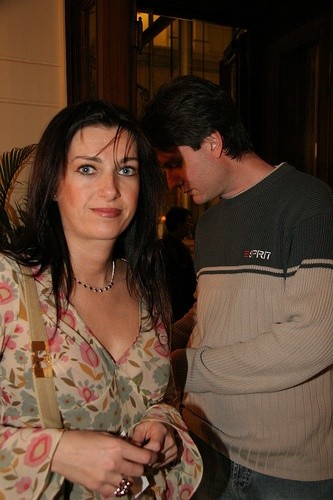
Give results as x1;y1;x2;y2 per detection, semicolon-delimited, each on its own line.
137;76;332;500
1;99;203;500
159;206;197;323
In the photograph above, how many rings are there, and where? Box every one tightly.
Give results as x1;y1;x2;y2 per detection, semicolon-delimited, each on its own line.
114;479;133;497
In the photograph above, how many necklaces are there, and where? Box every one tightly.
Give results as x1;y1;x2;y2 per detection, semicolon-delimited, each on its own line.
73;257;115;293
